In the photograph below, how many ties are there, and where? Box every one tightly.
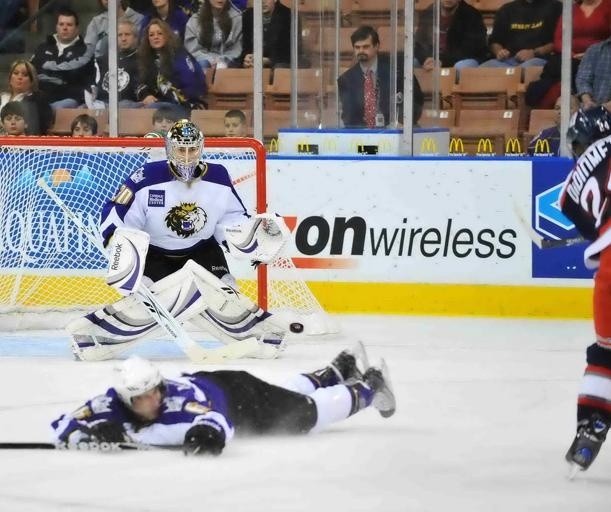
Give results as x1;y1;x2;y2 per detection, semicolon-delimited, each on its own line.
363;67;379;130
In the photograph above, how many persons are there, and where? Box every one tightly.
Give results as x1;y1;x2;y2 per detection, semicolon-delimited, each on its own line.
559;102;611;475
63;118;285;362
46;346;400;460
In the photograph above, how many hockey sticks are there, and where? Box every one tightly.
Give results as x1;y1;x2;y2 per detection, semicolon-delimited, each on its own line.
512;205;586;249
39;180;259;363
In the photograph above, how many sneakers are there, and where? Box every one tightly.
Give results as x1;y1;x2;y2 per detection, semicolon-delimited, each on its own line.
331;351;363;381
361;366;396;418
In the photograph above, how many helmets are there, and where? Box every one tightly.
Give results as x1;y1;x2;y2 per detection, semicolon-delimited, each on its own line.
165;118;205;185
565;103;611;158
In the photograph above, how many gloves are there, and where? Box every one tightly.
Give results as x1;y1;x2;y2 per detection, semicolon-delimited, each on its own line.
183;423;225;456
89;422;130;451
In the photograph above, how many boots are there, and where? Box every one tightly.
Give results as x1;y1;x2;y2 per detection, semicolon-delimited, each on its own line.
565;405;609;470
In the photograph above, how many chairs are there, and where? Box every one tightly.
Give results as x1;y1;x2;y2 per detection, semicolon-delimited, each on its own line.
1;0;611;160
